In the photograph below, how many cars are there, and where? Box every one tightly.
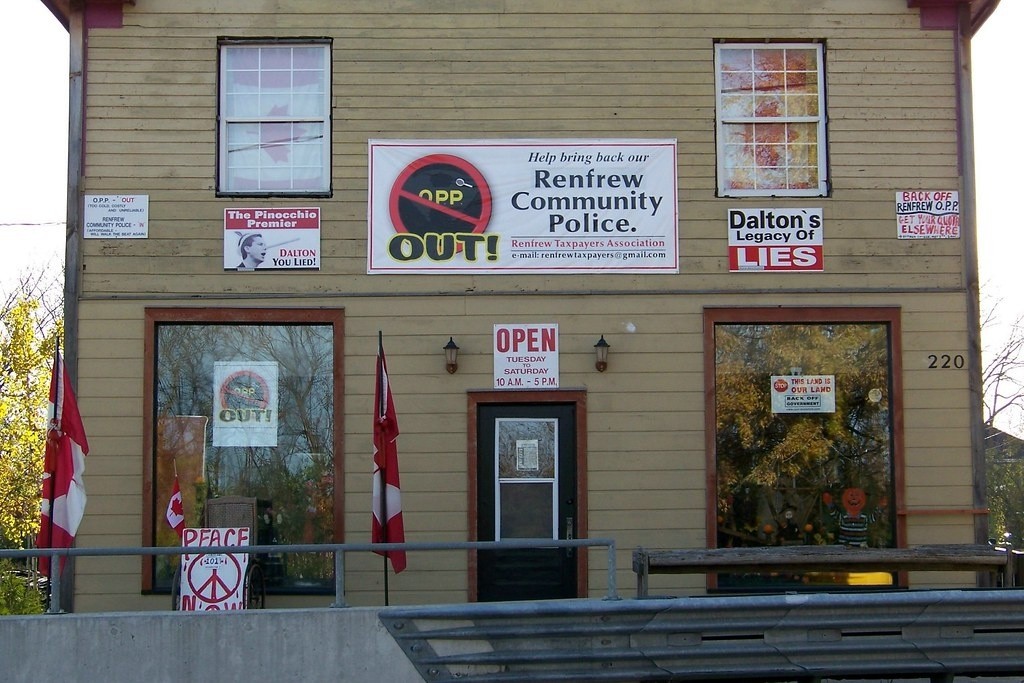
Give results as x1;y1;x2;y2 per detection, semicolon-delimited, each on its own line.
1;568;51;600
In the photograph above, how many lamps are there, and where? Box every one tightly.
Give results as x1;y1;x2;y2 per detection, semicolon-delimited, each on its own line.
443;336;460;374
594;334;611;372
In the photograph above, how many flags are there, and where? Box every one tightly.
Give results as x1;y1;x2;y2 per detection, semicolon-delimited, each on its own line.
34;356;89;578
166;478;185;536
370;347;406;573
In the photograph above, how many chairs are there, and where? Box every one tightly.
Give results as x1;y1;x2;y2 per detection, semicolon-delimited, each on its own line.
169;495;264;610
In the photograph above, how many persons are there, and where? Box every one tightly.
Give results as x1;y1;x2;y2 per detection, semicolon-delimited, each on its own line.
237;233;266;267
